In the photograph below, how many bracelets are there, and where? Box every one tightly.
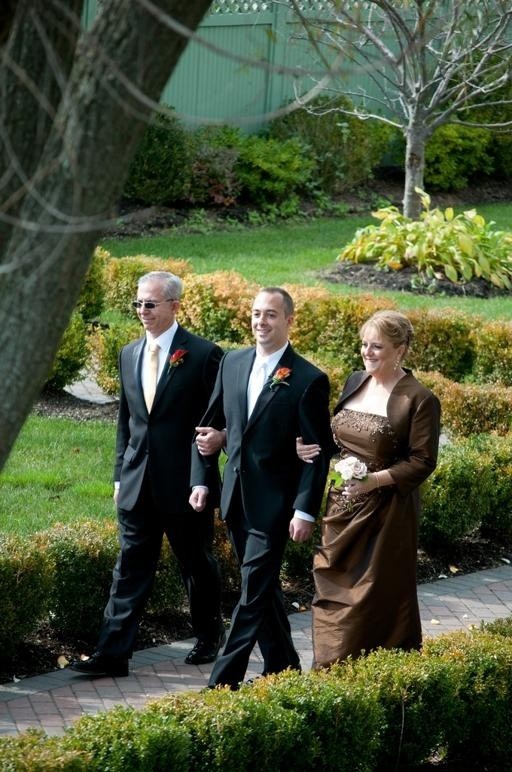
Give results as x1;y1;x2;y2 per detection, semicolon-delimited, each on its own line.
375;473;381;490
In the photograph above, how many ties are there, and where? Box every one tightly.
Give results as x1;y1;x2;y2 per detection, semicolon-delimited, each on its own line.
246;360;268;420
142;344;161;417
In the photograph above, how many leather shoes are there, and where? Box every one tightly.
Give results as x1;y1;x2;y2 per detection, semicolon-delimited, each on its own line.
68;649;132;677
183;628;230;665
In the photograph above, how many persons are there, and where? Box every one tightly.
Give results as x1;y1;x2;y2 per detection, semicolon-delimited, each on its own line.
185;285;335;692
70;267;228;678
294;308;442;675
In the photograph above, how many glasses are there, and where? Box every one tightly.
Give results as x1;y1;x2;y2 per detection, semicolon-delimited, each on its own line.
132;297;173;309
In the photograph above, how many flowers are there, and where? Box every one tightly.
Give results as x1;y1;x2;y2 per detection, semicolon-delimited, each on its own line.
167;349;187;374
267;367;294;392
328;455;368;512
